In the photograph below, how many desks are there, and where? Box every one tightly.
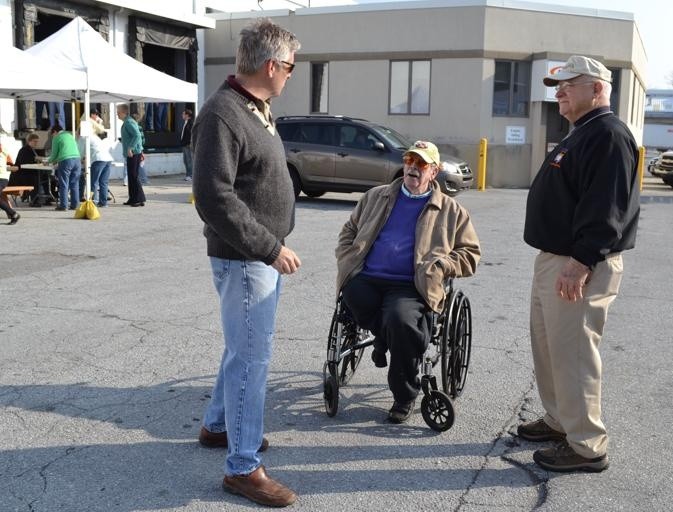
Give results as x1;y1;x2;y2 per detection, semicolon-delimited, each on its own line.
20;162;124;207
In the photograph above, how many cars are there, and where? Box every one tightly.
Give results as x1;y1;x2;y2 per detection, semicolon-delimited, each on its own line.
645;156;658;173
272;112;478;208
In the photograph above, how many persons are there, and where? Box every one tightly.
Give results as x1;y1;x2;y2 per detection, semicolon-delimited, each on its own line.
189;17;300;507
114;102;146;206
510;55;640;473
178;110;194;184
120;113;149;186
0;106;113;225
331;140;481;431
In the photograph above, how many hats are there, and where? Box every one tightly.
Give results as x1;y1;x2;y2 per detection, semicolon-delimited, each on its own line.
543;56;611;86
91;108;100;114
403;140;440;166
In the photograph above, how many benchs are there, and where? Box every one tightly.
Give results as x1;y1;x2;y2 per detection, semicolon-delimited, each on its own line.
1;186;34;208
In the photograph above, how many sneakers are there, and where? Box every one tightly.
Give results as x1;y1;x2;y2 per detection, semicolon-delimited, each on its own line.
389;401;415;422
517;419;609;472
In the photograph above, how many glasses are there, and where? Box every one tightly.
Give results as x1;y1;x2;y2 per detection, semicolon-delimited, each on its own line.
281;61;294;72
403;155;429;168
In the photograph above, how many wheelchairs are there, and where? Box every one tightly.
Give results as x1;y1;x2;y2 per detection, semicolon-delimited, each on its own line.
318;274;475;438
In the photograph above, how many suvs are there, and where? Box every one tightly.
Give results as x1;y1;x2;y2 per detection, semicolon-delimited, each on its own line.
646;150;673;186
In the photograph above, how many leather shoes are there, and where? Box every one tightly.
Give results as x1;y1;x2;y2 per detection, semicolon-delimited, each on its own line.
9;214;20;225
200;427;297;506
